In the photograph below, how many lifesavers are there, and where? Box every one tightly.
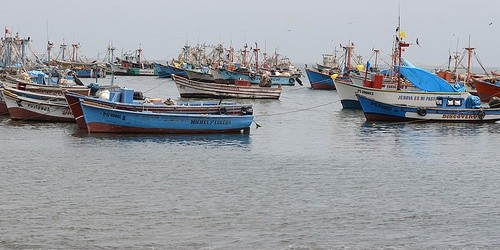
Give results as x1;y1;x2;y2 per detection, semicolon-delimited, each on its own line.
418;109;427;116
289;78;295;83
219;108;227;116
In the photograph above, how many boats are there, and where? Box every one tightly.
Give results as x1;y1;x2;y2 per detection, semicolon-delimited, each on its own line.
64;91;242;130
0;26;500;124
79;98;254;134
